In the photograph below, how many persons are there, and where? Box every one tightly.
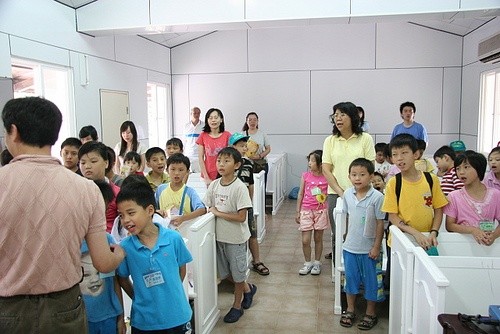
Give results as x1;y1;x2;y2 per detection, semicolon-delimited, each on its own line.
0;149;14;166
181;108;271;275
80;232;127;334
445;150;500;245
203;147;257;323
483;147;500;190
381;134;449;304
79;253;105;296
296;150;330;275
0;96;126;334
433;145;462;195
110;174;167;334
115;181;193;334
155;152;207;226
340;158;386;330
60;124;207;233
322;102;435;266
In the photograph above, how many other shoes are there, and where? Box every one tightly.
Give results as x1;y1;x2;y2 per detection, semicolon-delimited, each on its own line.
299;264;313;275
325;252;332;259
311;266;321;275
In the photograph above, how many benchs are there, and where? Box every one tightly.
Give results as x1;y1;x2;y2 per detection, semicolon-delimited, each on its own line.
124;153;287;334
333;196;500;334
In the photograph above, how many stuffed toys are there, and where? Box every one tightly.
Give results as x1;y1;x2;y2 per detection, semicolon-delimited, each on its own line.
245;141;258;158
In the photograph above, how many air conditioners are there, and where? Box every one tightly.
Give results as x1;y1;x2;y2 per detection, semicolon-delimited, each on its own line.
477;30;500;64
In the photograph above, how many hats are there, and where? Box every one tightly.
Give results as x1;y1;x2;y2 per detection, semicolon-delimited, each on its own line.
228;132;250;146
449;140;465;151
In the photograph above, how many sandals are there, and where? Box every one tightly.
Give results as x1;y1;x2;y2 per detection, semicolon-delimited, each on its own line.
241;283;257;309
339;310;355;328
252;261;270;276
223;307;244;323
356;314;378;330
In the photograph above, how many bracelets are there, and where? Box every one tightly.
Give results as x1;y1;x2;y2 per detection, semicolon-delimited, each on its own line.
259;154;261;158
430;230;437;236
